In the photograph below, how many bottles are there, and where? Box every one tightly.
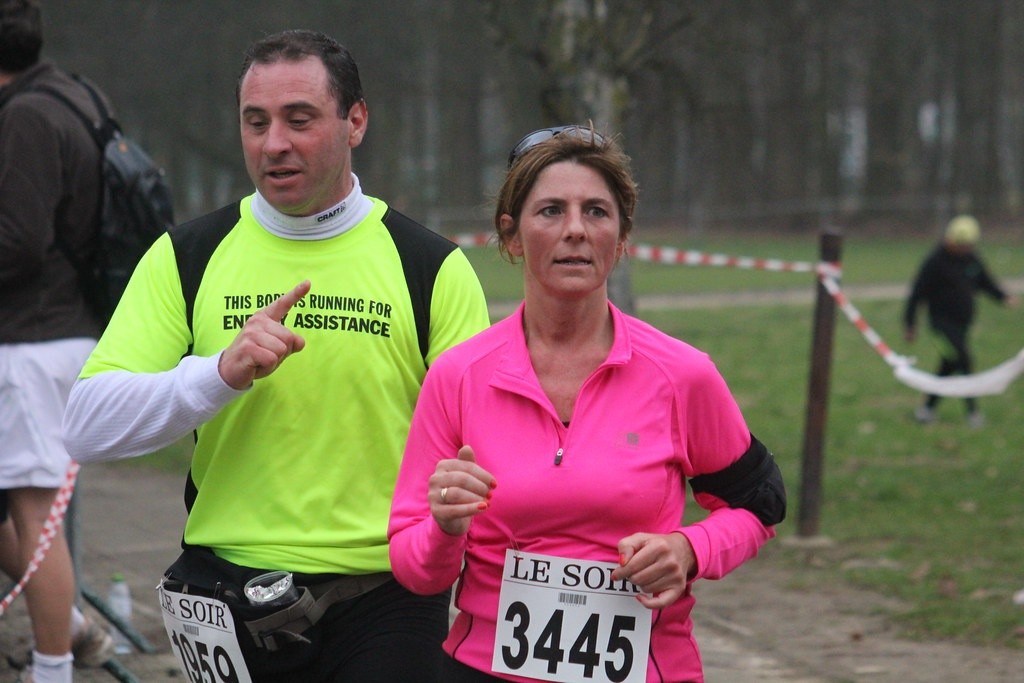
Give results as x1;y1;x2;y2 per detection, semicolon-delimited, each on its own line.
110;575;132;655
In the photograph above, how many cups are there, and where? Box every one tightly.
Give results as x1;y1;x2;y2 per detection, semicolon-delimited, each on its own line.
244;571;297;605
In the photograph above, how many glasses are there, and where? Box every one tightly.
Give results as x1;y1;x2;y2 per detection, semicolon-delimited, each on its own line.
507;125;609;173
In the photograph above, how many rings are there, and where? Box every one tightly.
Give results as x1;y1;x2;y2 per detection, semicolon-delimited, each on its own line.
440;488;448;504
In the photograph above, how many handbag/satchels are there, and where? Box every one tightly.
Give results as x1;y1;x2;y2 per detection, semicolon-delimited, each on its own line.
0;70;175;328
160;551;314;683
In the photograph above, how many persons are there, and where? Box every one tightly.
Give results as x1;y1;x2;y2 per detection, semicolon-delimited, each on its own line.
0;1;114;683
387;125;787;683
901;214;1017;426
58;30;491;683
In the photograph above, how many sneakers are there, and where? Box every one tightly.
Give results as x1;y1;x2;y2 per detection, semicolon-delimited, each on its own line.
7;615;115;670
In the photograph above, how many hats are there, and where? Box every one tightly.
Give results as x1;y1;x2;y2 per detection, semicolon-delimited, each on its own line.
946;215;981;245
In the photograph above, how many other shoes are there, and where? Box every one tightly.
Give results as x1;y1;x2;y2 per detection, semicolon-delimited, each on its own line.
965;413;982;431
916;407;936;425
